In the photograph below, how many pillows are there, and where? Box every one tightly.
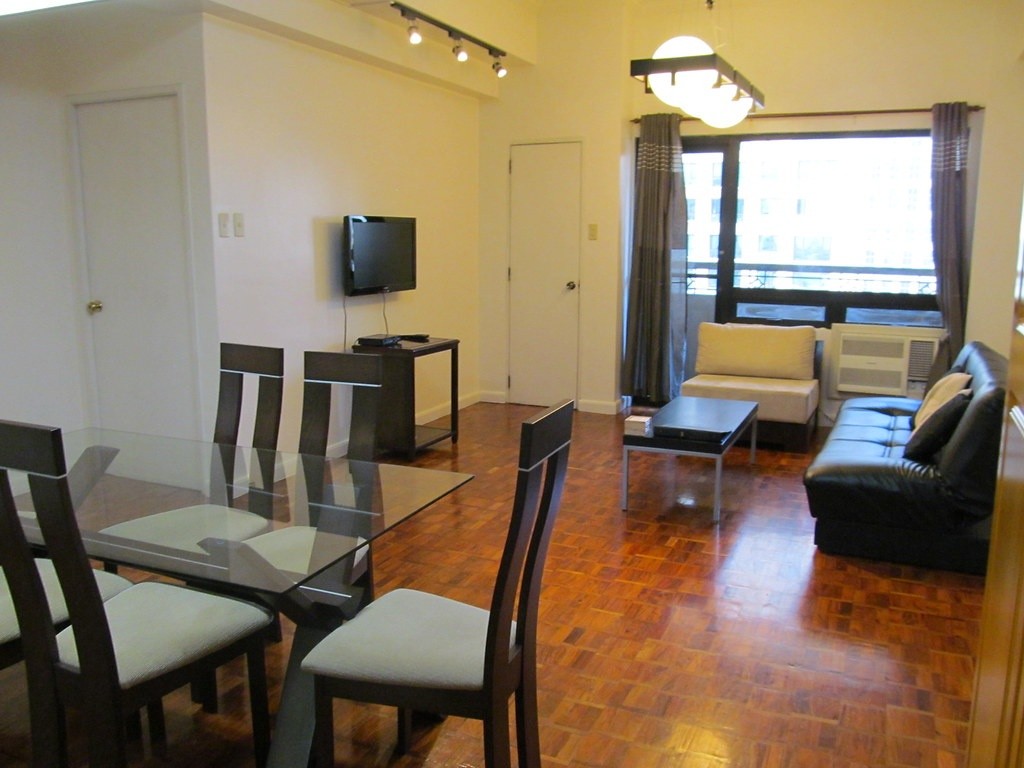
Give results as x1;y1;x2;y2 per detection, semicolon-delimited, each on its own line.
902;338;972;461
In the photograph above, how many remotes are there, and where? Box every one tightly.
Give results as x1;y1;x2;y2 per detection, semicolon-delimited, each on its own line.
398;334;430;340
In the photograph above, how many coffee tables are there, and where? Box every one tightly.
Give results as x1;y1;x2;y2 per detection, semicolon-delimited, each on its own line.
622;395;759;521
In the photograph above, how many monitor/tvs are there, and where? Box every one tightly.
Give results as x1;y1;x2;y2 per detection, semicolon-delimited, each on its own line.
344;215;416;296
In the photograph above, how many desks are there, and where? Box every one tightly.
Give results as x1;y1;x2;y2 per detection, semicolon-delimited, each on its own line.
352;337;459;462
6;425;474;706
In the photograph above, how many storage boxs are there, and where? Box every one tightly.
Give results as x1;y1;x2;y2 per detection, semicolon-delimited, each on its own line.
624;415;651;427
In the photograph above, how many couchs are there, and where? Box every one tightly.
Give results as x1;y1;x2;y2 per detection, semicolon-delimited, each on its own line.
680;322;824;454
802;342;1008;576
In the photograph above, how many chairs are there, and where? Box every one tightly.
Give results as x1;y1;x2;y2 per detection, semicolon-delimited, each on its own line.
0;340;388;768
299;398;576;768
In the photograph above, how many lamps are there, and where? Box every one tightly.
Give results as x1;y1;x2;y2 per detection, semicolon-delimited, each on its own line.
627;35;764;128
389;2;506;79
